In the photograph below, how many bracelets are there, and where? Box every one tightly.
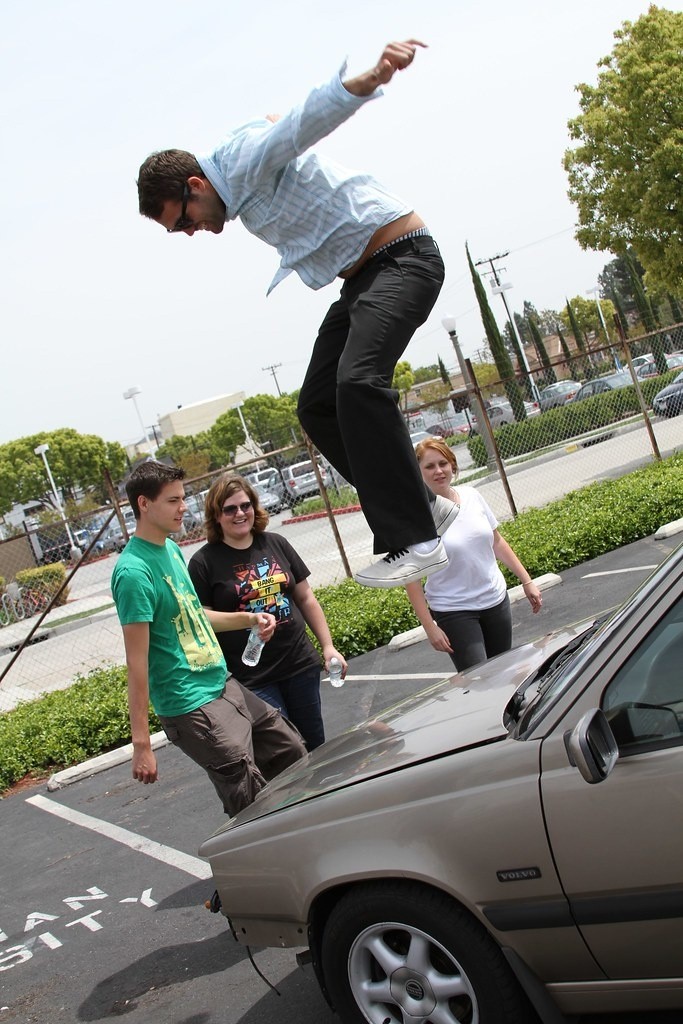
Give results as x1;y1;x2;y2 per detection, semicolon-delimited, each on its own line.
523;580;532;586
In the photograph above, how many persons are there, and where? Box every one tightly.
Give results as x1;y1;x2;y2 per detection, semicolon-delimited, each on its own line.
112;461;308;818
188;473;349;752
407;437;542;674
137;38;459;586
450;632;553;691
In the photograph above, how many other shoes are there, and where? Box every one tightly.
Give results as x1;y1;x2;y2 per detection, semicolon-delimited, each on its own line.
209;891;221;914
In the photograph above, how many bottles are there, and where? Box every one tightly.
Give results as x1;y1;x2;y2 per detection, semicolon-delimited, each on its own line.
328;657;345;688
242;618;268;667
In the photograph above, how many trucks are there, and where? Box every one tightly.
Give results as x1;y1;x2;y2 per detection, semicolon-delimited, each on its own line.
40;528;89;560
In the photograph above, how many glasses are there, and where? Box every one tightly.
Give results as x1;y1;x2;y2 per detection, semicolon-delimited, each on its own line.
221;502;252;517
167;184;194;233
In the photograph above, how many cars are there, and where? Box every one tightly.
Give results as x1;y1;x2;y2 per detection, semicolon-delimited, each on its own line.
83;482;281;553
411;432;446;451
197;556;683;1024
652;370;683;418
533;379;582;409
475;396;540;432
624;350;683;377
565;373;647;405
427;411;477;437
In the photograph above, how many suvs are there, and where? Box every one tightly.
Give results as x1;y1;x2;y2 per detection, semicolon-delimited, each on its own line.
247;468;280;487
265;459;327;505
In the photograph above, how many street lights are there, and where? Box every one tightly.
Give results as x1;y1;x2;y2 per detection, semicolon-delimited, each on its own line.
35;443;82;561
587;284;620;371
121;386;156;459
230;399;260;472
440;313;504;471
491;283;535;388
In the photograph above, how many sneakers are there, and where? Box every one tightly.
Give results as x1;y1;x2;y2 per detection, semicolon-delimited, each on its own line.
354;535;450;588
431;494;460;537
